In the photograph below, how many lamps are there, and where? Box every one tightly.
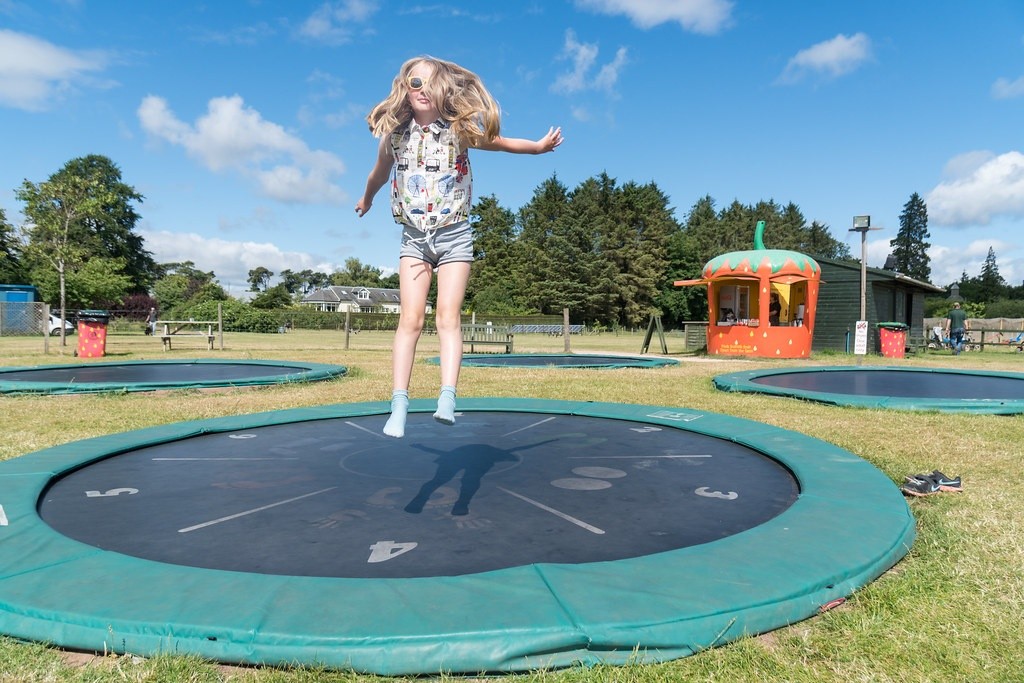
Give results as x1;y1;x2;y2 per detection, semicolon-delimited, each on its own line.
852;216;870;228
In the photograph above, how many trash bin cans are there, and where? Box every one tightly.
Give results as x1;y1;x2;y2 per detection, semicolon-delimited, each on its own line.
876;321;910;359
74;310;111;357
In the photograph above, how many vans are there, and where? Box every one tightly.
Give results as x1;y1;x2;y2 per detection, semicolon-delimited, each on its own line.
20;309;74;336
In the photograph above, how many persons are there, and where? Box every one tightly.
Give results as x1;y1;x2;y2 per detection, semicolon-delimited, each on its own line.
946;302;969;355
145;307;159;336
769;293;781;326
356;56;565;439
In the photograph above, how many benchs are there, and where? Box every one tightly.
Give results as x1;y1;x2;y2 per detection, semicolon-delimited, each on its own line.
461;324;513;355
153;333;215;351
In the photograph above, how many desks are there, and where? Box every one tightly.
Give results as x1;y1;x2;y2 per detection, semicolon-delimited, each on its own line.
155;320;219;350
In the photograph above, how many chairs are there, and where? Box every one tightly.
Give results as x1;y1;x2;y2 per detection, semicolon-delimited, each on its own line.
905;329;926;353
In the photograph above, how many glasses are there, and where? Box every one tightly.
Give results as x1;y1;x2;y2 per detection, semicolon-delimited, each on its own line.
408;77;428;89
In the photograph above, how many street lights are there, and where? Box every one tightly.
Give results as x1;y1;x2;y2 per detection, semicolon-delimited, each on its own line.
848;215;870;322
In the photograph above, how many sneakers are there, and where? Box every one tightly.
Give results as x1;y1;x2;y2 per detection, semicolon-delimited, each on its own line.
899;475;940;496
931;470;965;492
